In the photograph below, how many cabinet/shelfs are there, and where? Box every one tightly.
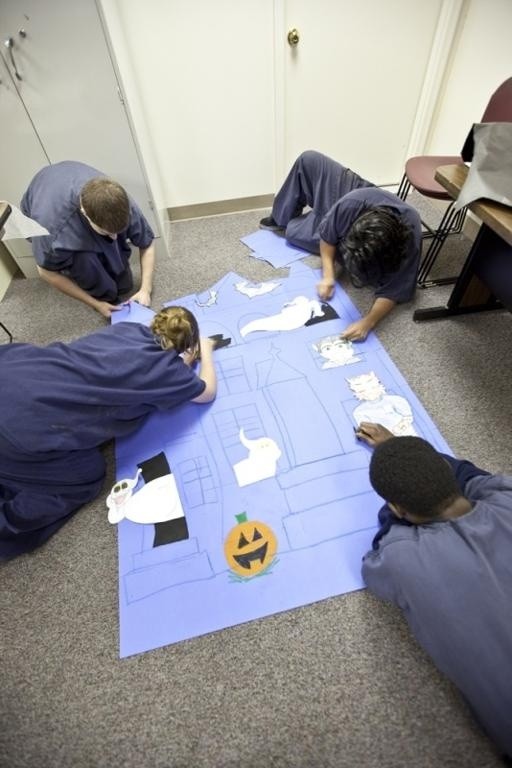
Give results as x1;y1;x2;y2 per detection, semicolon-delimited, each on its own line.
0;0;172;277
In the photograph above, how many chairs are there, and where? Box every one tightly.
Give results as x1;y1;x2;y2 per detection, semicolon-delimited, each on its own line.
398;74;512;290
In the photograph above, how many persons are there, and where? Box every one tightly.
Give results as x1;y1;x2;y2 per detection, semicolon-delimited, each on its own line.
256;146;426;345
0;304;218;569
19;159;159;322
351;416;512;768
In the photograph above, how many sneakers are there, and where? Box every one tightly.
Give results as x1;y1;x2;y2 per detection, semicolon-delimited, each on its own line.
260;216;282;231
